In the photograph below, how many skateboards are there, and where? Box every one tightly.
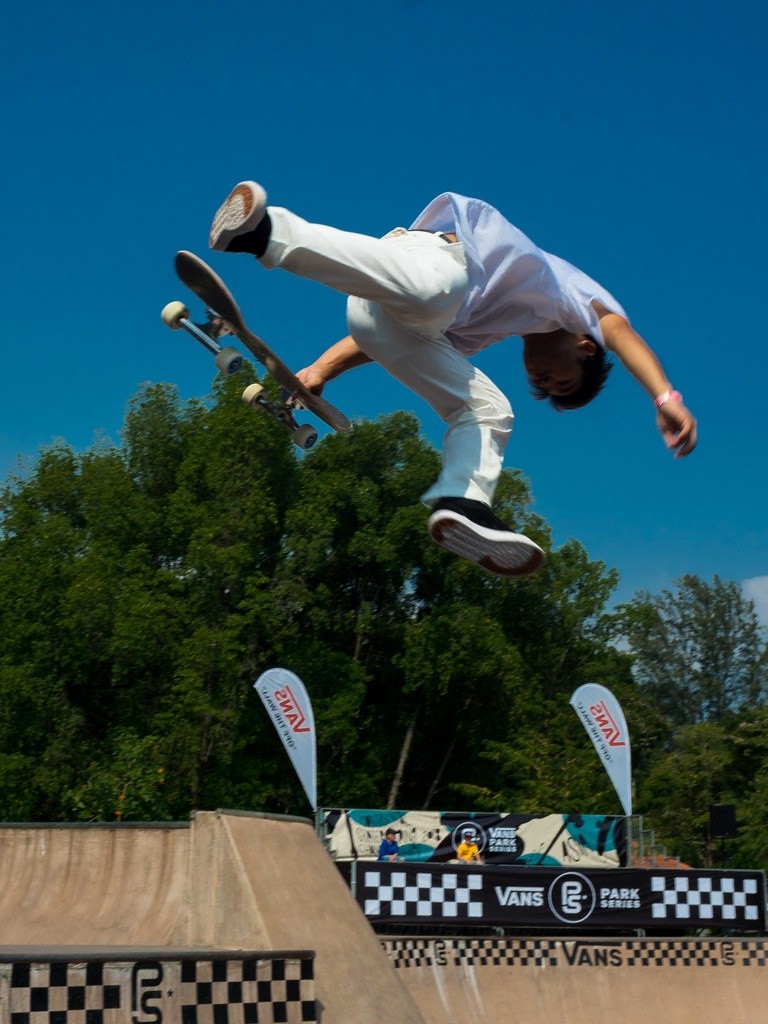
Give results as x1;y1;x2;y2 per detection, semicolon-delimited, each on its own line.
160;249;352;449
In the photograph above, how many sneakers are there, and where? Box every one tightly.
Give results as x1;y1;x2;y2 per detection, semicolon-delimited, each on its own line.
426;498;546;577
209;180;270;255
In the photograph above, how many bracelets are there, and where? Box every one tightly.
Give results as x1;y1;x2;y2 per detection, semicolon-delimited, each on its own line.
653;389;684;410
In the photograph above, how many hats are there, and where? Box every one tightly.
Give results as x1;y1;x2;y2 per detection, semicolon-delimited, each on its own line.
386;828;397;835
465;833;473;838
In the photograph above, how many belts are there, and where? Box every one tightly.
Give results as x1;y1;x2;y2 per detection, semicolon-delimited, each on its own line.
411;229;458;245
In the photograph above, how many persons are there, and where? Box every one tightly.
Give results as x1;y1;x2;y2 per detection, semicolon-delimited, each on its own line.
378;828;405;863
456;831;485;865
208;179;698;579
